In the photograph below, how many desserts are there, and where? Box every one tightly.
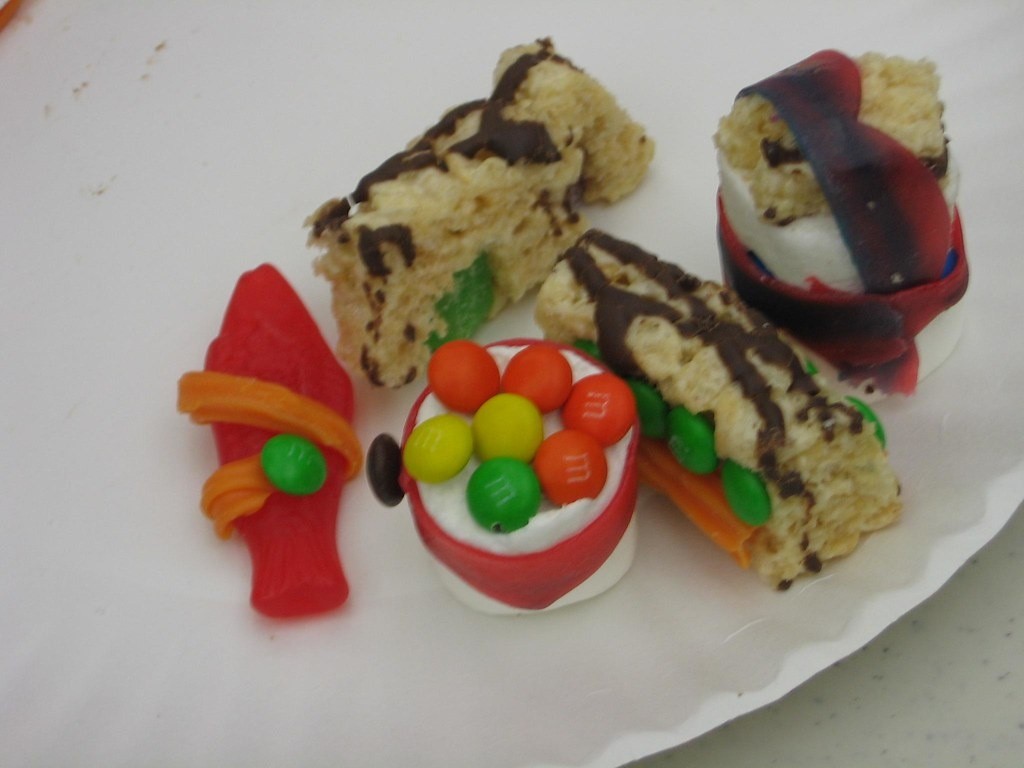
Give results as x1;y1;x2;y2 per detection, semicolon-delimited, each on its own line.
366;338;639;614
715;50;971;397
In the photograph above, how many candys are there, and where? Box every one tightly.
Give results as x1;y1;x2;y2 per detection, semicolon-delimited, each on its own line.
177;263;361;617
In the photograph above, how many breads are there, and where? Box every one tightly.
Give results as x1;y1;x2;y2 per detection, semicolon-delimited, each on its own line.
534;229;902;584
304;38;656;387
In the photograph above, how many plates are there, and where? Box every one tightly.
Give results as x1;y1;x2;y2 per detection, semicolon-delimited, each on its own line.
4;4;1024;767
